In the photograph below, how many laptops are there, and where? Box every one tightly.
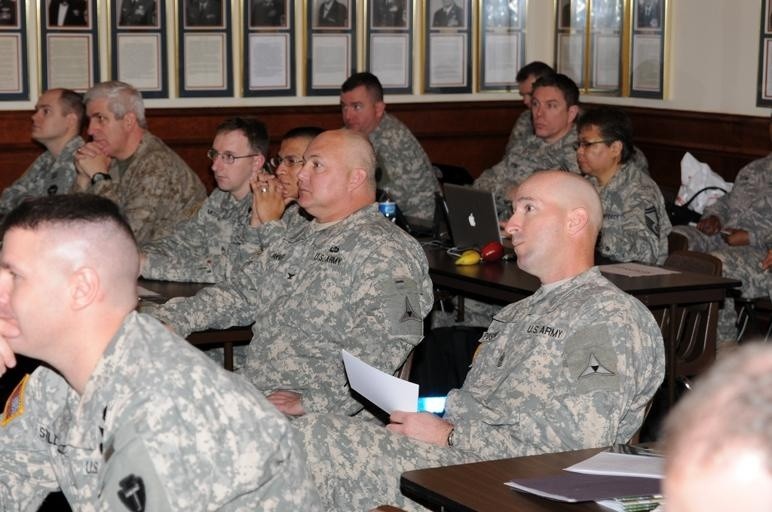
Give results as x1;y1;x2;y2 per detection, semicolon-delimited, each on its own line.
442;182;517;256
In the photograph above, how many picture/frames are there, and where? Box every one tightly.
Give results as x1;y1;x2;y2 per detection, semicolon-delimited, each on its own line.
174;0;234;99
304;0;357;97
756;0;772;107
107;0;169;100
240;0;297;98
421;0;473;94
586;0;624;97
475;0;528;94
363;0;413;95
553;0;589;95
628;0;667;100
36;0;101;97
0;1;32;101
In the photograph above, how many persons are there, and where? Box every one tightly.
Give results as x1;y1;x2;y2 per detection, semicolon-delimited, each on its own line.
0;86;86;217
0;192;319;512
572;115;672;268
340;72;443;221
472;73;583;235
138;128;433;425
136;115;273;284
655;341;772;511
72;80;207;251
670;154;770;251
711;244;770;366
290;173;665;511
505;62;555;153
230;131;321;290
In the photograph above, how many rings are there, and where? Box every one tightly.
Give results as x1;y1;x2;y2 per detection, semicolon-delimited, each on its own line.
261;187;268;193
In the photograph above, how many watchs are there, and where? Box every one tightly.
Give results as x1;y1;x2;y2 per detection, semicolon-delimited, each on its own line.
446;429;453;446
89;172;112;185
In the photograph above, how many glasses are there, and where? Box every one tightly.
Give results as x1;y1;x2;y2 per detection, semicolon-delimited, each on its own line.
206;148;259;165
269;155;304;168
570;139;608;151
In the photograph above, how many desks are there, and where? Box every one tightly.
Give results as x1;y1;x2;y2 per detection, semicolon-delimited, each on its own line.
134;277;254;372
394;214;742;448
399;443;672;511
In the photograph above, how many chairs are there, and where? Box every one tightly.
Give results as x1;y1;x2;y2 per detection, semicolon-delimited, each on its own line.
399;324;492;399
632;249;723;375
